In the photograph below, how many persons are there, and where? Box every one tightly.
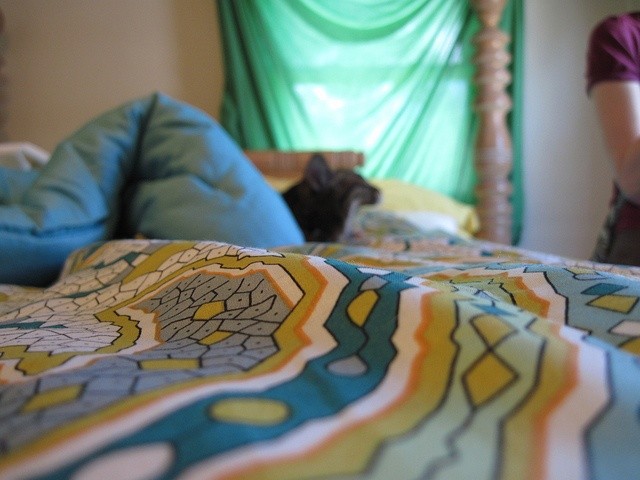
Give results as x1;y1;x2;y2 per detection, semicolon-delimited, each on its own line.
585;10;638;271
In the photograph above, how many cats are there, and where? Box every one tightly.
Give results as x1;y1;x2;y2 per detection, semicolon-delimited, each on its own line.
281;151;382;242
